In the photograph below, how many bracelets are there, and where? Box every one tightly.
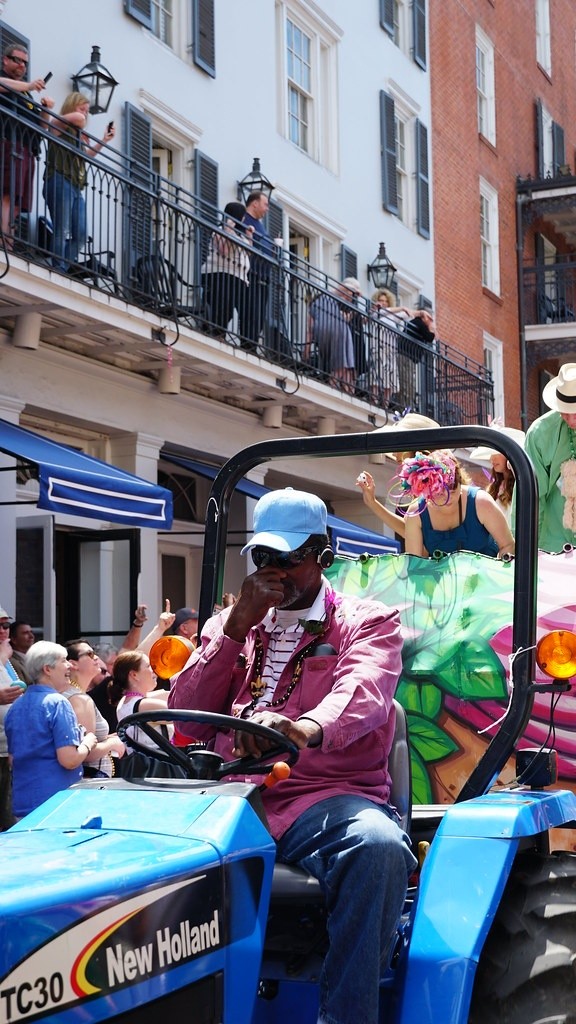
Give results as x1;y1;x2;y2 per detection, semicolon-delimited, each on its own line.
81;743;91;756
133;620;145;628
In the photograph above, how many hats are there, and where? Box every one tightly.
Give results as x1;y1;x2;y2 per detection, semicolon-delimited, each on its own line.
171;608;199;632
542;363;576;414
240;486;327;555
470;415;525;460
366;407;440;460
0;608;8;618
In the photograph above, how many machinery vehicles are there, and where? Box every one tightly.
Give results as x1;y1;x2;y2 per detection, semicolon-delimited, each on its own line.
0;425;576;1022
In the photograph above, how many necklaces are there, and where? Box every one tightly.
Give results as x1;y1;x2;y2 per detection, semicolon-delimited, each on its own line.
250;633;326;709
70;679;85;693
7;658;21;680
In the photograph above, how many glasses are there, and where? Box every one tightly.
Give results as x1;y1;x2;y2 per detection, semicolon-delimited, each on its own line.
7;55;29;68
252;545;322;570
77;650;97;658
0;622;11;630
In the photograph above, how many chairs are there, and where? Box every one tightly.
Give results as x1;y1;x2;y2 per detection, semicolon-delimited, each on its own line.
538;293;574;323
273;325;321;378
438;400;464;427
272;698;414;902
34;213;119;296
136;256;212;335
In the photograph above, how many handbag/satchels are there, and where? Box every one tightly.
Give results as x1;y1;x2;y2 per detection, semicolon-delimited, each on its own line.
117;697;187;779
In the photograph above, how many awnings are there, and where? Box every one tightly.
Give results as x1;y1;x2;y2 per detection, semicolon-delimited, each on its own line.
158;452;401;557
0;417;173;530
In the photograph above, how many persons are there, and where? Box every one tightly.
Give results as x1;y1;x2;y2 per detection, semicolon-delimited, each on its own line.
235;192;278;352
304;284;362;392
42;92;116;274
0;43;47;251
0;608;27;831
396;305;437;410
470;363;576;557
201;202;255;340
3;639;98;824
334;277;374;397
9;621;36;685
356;412;515;559
167;487;418;1024
88;596;199;778
367;287;434;409
63;641;126;779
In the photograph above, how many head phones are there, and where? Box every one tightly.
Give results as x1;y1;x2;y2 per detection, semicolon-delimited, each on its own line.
317;546;334;570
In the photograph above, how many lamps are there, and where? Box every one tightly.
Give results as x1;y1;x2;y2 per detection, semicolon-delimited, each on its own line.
316;417;335;435
263;406;282;429
369;453;385;466
156;367;183;395
10;312;43;349
72;46;118;114
369;241;396;287
235;156;275;208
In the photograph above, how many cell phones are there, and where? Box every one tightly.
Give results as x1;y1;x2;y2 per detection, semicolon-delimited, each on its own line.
44;72;53;84
108;121;114;135
